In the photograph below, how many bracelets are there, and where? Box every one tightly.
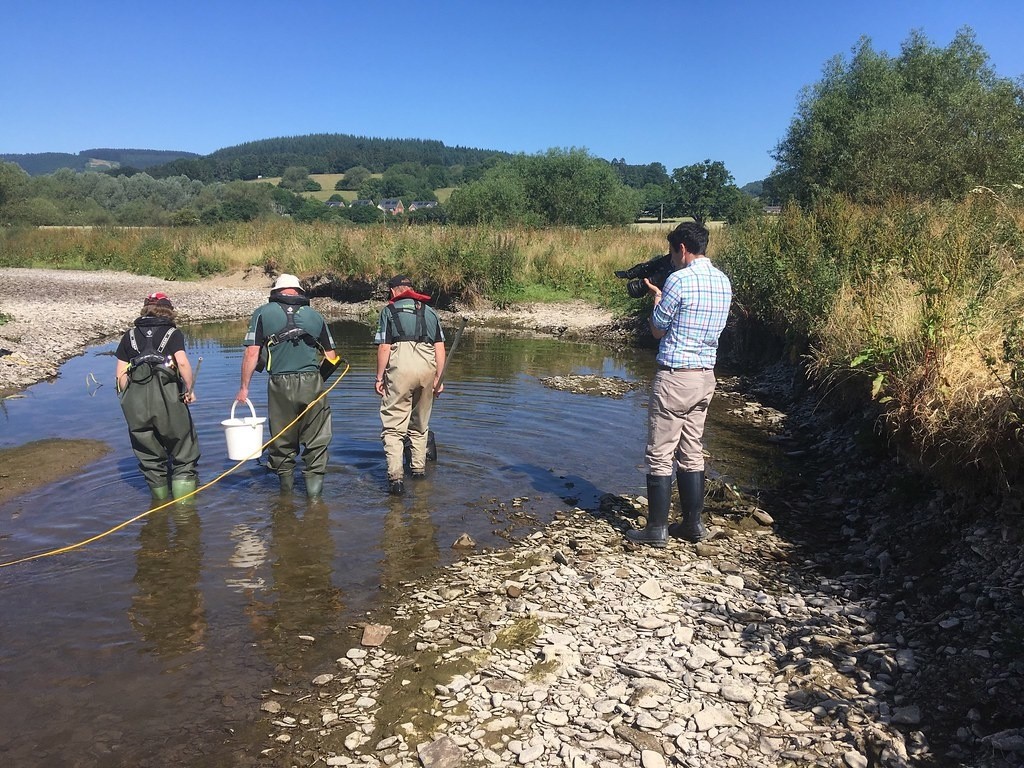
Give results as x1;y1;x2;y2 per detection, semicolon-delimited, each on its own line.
375;377;383;382
654;293;663;297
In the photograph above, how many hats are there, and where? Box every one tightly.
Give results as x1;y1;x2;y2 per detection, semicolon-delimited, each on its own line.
270;274;305;296
144;293;174;310
389;285;431;304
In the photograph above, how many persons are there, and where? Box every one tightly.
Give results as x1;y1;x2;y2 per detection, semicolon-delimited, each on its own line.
626;223;732;548
247;497;345;680
377;480;440;597
235;274;341;498
115;293;201;503
129;500;206;676
373;275;446;496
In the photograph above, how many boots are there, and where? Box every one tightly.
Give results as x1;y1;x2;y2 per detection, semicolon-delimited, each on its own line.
626;473;671;548
306;474;324;499
151;484;167;500
669;471;706;542
172;480;196;503
279;474;293;492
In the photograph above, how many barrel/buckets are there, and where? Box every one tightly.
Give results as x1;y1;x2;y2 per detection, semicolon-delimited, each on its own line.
220;398;267;460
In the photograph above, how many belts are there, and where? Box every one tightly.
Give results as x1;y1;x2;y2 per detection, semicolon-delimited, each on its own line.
658;363;710;372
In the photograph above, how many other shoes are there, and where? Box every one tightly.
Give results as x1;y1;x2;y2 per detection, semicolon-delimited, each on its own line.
392;482;404;496
413;472;426;478
